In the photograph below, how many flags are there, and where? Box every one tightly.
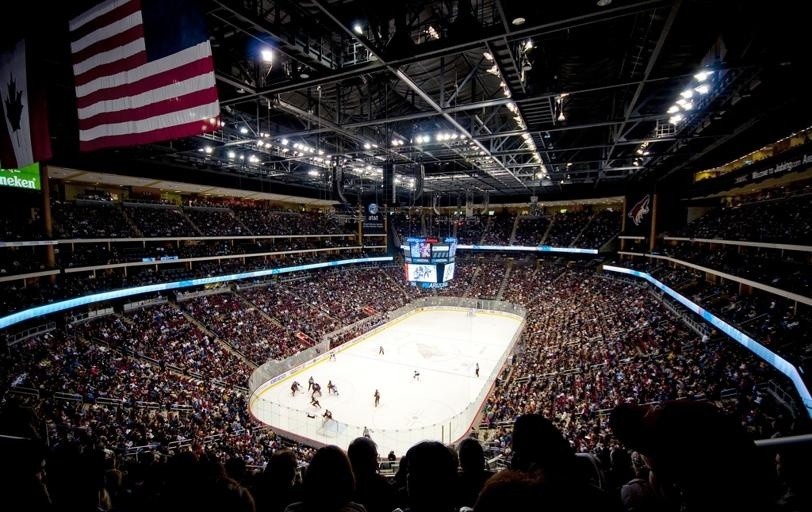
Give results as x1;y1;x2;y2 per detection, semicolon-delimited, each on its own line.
67;0;223;151
0;21;53;169
